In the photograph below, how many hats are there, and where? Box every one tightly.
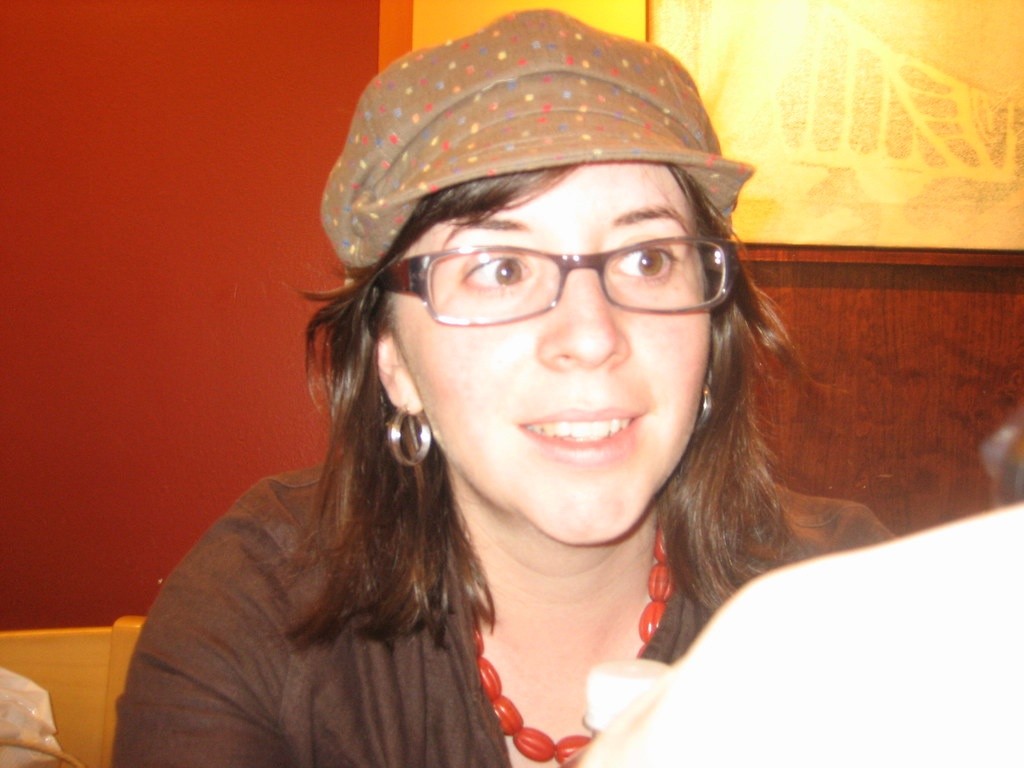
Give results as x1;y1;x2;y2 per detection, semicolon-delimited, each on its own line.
321;8;756;309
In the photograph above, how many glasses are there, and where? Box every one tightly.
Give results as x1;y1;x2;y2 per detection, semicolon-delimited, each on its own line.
373;236;737;327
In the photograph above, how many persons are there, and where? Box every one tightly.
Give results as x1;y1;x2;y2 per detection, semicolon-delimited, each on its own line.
578;500;1023;768
108;5;891;768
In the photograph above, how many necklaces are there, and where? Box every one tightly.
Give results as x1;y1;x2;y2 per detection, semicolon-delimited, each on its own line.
471;531;674;767
